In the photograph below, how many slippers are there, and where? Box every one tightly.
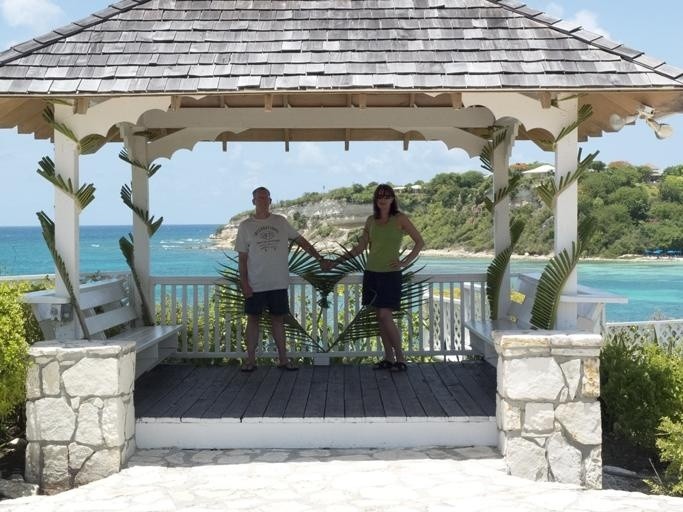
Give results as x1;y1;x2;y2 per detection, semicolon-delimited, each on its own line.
277;364;298;370
241;363;255;371
372;360;393;370
391;362;406;372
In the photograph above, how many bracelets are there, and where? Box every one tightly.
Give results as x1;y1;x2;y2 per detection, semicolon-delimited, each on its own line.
318;257;322;261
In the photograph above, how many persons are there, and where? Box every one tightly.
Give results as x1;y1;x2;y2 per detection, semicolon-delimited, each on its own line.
322;183;425;374
233;187;327;371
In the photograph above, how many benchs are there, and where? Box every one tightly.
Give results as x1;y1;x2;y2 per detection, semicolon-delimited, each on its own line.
22;279;183;383
465;277;538;371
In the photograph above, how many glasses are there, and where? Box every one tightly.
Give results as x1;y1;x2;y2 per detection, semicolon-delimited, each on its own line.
378;194;391;199
